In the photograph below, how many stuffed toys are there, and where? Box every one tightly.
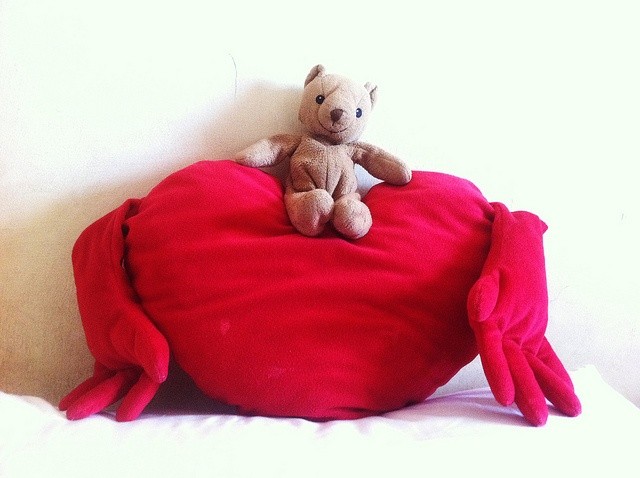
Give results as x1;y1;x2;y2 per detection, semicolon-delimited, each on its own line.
235;64;412;240
